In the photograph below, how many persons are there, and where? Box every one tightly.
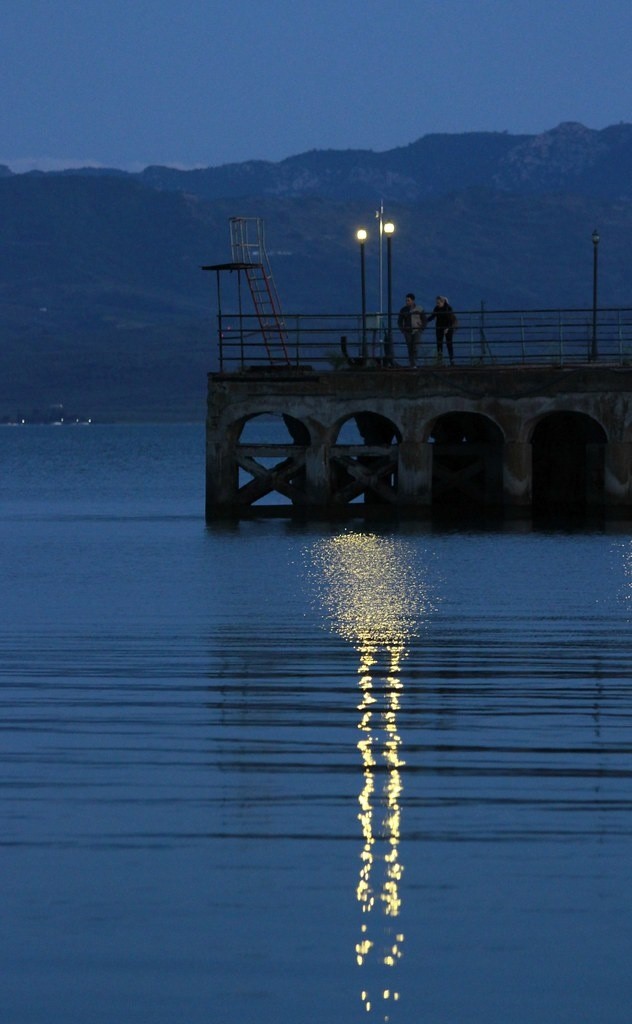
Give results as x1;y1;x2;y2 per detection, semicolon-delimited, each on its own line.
426;296;457;364
398;293;424;368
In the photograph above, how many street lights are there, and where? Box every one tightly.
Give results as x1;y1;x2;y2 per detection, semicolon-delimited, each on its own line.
384;222;395;328
356;228;367;329
592;229;600;340
